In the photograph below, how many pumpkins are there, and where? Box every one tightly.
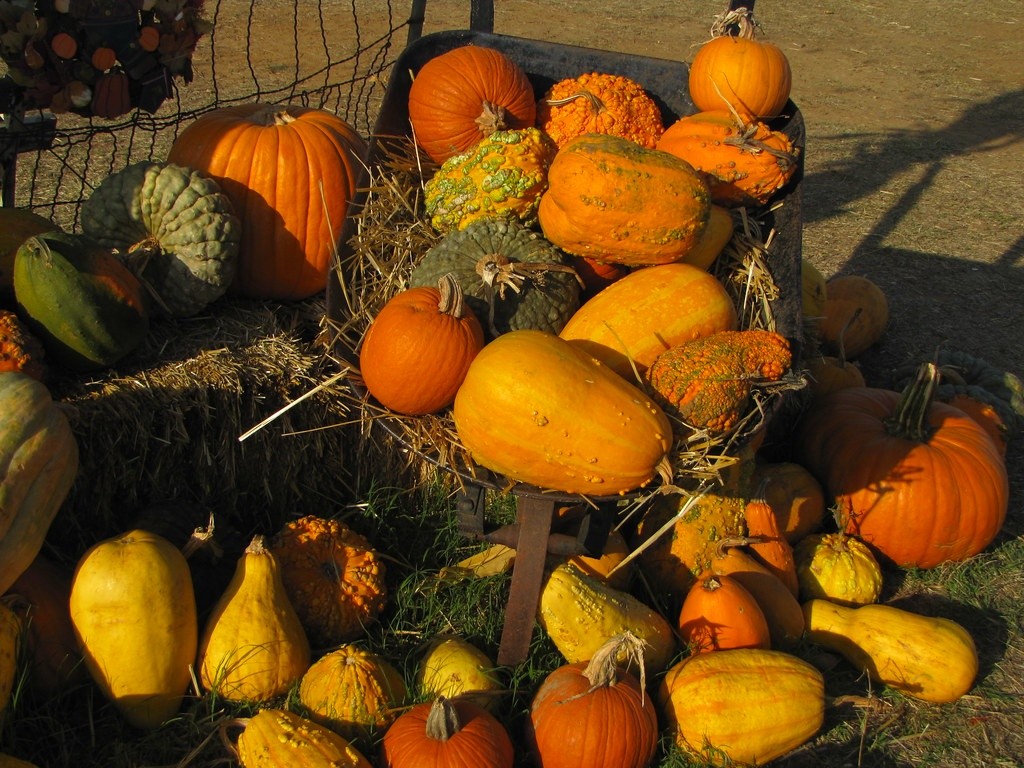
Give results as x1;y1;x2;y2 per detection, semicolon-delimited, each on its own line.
0;12;1005;768
